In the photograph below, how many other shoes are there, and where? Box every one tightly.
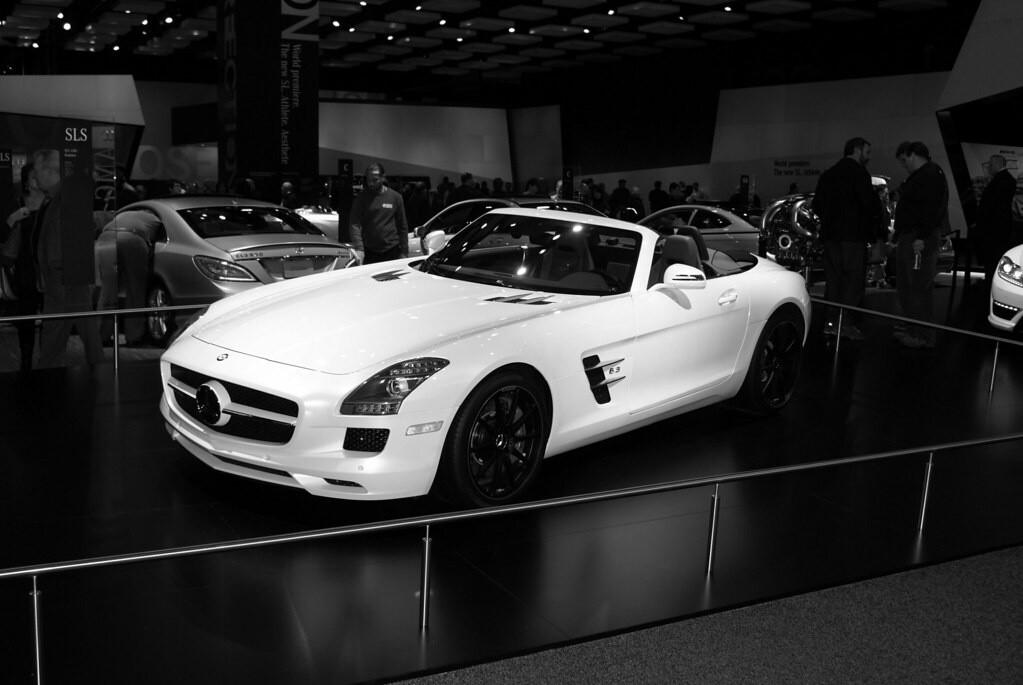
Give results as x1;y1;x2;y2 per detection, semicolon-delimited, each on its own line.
101;336;115;347
126;337;149;348
901;333;937;348
20;362;32;382
823;318;866;340
893;323;909;337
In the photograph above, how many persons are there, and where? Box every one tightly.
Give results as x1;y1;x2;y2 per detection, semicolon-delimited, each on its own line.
277;182;297;209
165;176;262;201
552;177;631;216
93;208;165;347
977;155;1017;284
810;136;952;348
0;149;112;385
438;172;548;209
113;161;146;207
789;182;801;194
383;178;442;232
349;163;409;266
648;180;669;213
669;181;706;205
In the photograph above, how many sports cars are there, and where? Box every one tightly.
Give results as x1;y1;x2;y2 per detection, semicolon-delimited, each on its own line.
159;206;813;515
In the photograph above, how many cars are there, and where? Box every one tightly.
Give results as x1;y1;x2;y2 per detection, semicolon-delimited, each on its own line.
621;203;768;252
92;196;366;345
1012;186;1023;217
985;242;1023;342
406;195;618;260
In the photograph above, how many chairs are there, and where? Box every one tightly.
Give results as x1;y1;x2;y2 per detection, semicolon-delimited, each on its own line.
650;235;702;286
540;230;594;277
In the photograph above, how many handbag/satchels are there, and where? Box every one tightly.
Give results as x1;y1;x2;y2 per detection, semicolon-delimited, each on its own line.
0;197;23;266
